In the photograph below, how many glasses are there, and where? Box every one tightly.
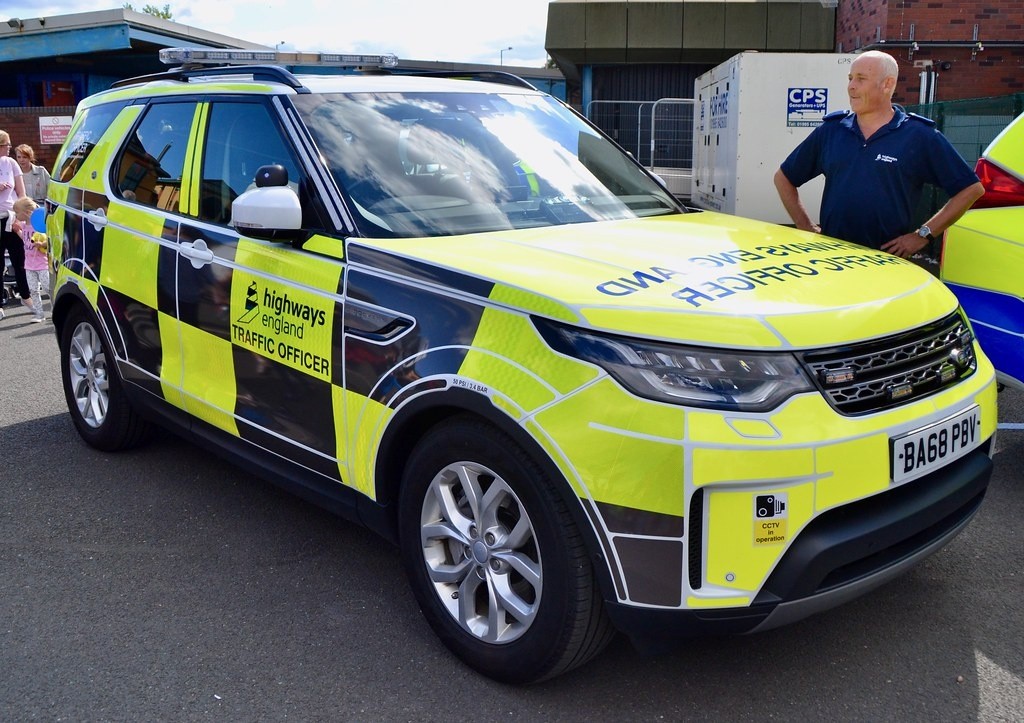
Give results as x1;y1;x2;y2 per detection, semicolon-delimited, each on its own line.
0;143;12;148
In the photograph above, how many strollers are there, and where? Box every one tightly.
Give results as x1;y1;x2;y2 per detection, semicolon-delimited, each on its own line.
2;198;46;303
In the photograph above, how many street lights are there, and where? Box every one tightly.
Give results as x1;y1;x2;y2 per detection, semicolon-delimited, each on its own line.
501;47;512;65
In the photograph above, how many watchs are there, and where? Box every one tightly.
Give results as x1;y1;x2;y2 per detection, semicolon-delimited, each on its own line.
917;224;934;241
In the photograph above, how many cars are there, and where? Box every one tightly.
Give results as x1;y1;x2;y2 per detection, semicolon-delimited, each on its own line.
398;117;540;199
938;108;1024;393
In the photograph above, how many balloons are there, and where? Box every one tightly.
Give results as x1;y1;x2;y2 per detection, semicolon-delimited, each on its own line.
31;207;47;232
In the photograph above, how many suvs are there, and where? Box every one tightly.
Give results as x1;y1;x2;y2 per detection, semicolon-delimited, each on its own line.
43;70;998;684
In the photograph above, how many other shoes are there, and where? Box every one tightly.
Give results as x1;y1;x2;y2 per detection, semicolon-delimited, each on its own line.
0;309;4;320
30;317;46;323
21;299;36;312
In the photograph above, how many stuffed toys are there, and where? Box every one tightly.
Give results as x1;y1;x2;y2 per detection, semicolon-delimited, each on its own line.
31;232;48;254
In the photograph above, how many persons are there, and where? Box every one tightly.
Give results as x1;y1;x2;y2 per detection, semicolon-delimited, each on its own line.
774;50;985;259
0;130;51;323
